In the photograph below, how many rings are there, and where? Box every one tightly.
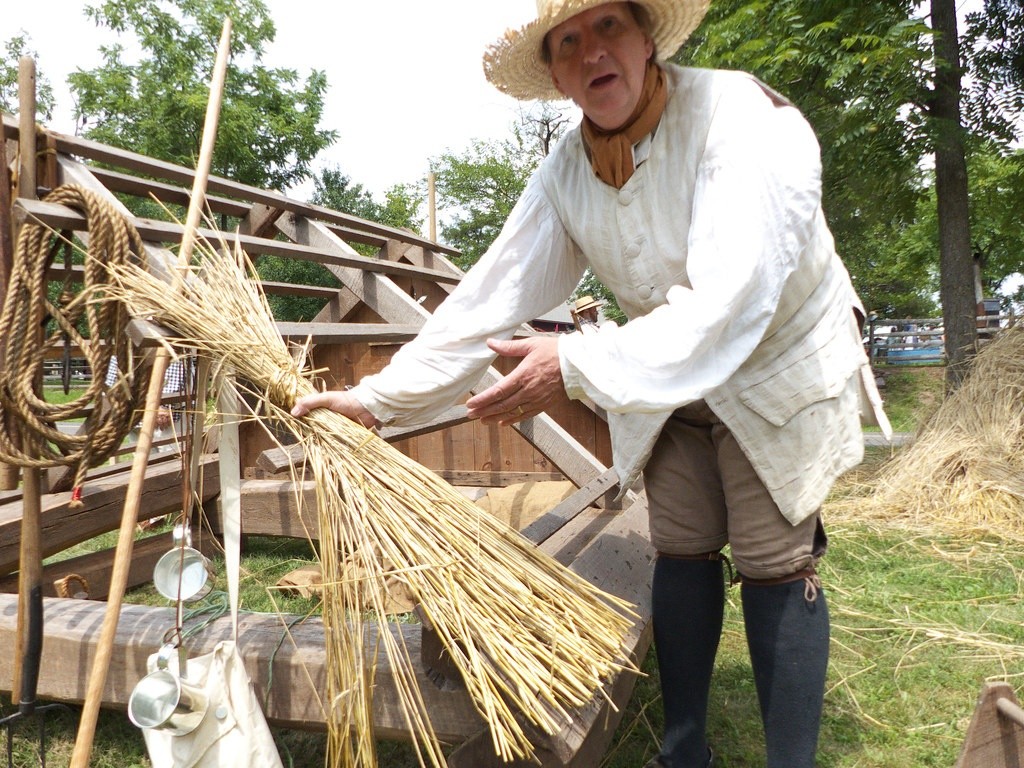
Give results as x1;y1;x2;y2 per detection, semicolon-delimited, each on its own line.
517;404;523;416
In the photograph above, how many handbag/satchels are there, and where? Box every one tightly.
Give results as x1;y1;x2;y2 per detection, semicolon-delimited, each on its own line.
141;641;284;768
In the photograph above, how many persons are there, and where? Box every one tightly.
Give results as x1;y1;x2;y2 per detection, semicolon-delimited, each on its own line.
288;0;891;768
570;296;604;329
104;348;196;453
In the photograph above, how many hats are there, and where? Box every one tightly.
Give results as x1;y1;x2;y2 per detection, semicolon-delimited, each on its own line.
482;0;710;102
570;296;604;315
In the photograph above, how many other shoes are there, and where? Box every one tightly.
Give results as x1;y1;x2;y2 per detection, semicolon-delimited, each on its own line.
643;744;713;768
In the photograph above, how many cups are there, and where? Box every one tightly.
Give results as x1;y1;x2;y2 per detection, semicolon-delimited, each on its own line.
128;647;210;738
152;519;215;603
